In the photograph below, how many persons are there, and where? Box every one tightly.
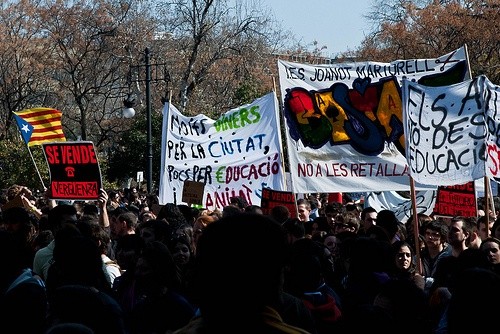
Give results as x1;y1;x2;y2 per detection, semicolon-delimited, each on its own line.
0;183;500;334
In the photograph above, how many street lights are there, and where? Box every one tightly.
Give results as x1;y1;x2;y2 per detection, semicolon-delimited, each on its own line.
122;46;172;194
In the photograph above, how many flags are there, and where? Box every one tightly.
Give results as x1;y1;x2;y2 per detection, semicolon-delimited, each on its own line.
11;107;68;148
